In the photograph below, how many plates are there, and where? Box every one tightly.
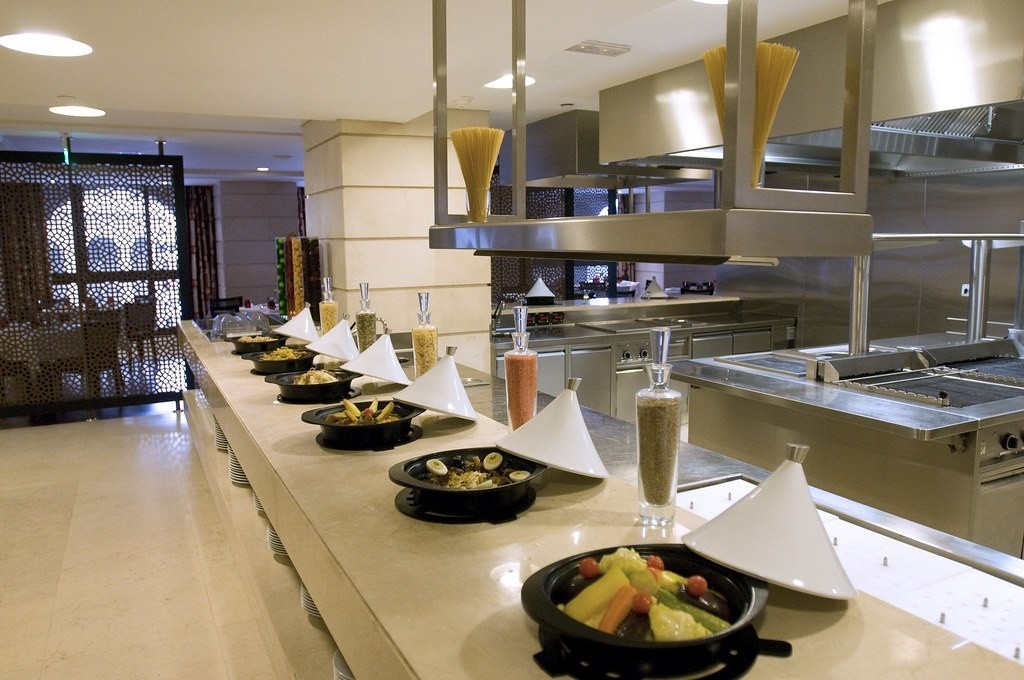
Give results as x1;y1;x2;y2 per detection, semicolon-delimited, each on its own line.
224;333;769;680
212;412;356;680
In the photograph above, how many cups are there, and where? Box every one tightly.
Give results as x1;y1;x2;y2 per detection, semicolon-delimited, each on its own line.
210;312;270;336
750;149;766;188
464;187;490;224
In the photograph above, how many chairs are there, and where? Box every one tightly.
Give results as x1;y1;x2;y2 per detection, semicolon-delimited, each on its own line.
1;295;159;414
210;296;243;318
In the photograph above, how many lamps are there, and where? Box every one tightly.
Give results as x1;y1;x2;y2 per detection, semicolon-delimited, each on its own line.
48;96;107;117
483;73;537;89
0;29;94;58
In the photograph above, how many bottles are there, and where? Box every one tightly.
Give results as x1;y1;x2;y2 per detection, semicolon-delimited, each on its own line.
412;291;438;380
503;305;538;435
634;327;685;529
318;277;338;336
355;281;377;355
